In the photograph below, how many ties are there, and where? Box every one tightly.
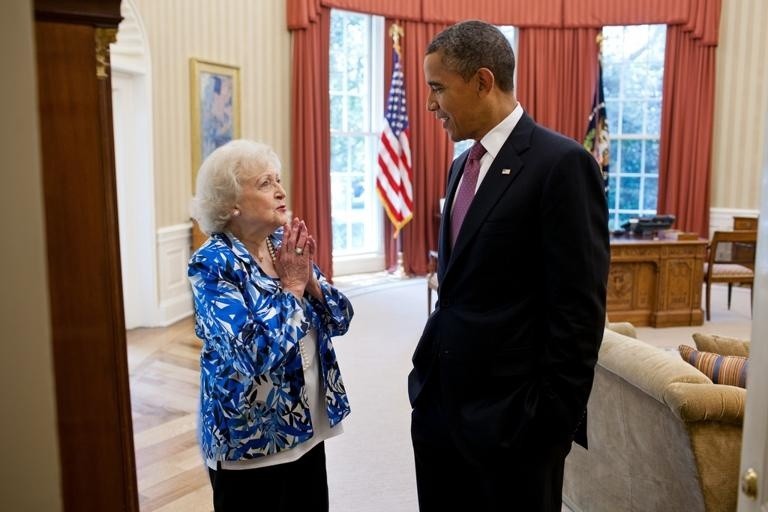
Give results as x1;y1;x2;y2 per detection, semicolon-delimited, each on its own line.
450;143;487;251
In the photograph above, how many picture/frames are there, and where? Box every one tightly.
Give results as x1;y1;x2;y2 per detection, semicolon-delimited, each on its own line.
190;58;244;196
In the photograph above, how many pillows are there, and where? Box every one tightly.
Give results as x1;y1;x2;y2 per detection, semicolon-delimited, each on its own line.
690;331;749;357
678;342;749;389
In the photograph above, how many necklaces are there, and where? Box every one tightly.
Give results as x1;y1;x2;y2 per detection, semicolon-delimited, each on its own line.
265;236;278;265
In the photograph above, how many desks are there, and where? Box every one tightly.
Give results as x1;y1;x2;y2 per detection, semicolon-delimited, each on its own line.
600;227;709;327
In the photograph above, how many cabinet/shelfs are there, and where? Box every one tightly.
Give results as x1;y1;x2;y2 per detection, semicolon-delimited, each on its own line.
729;215;757;289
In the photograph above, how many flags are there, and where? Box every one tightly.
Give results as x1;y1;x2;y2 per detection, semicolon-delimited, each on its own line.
374;25;416;236
581;54;613;196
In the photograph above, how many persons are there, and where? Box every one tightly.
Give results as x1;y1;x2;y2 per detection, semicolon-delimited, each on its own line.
408;18;612;510
182;139;354;509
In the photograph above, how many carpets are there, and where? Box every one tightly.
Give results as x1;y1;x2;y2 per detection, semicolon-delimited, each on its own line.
324;279;440;512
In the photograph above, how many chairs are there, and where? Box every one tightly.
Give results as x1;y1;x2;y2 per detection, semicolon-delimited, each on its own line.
701;229;756;321
426;249;438;316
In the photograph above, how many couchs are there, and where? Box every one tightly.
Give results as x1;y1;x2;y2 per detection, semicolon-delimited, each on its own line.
559;307;750;511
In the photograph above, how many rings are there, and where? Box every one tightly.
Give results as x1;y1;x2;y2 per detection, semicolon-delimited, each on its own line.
295;247;303;255
